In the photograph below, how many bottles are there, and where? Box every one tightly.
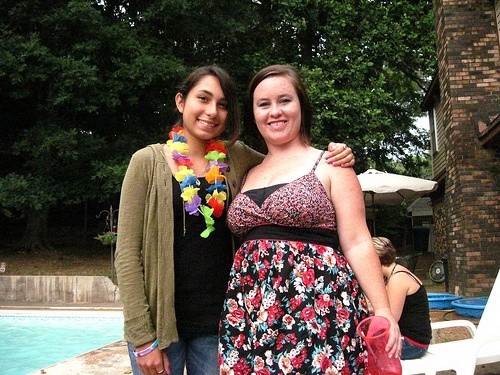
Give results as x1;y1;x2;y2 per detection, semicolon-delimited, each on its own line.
356;316;402;375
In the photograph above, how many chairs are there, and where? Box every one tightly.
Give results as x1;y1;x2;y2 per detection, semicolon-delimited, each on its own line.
400;268;500;375
396;249;423;272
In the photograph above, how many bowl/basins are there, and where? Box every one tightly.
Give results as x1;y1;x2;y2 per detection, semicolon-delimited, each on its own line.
426;292;462;309
451;297;490;318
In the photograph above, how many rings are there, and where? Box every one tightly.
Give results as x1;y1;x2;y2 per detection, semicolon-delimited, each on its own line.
399;337;404;340
157;370;163;374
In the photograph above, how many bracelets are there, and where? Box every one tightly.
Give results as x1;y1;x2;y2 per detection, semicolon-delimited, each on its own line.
133;339;158;357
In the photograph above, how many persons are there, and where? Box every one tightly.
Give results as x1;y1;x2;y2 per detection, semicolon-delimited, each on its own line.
216;64;403;375
371;237;432;360
114;66;356;375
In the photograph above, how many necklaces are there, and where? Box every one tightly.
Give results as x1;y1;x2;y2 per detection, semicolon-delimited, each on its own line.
384;262;397;286
166;125;229;238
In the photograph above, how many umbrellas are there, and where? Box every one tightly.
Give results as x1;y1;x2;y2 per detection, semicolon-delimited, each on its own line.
356;168;438;237
